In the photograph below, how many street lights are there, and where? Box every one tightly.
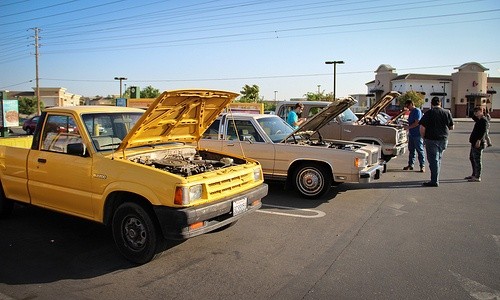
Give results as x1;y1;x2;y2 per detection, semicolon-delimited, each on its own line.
115;77;127;99
439;81;451;108
325;61;344;101
274;91;278;100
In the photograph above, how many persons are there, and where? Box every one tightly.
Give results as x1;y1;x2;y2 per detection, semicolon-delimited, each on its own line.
403;100;426;172
483;109;492;147
419;97;454;187
287;103;307;130
465;106;489;182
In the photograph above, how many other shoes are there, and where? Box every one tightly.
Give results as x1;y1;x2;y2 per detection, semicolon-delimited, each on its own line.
422;181;439;187
420;167;425;172
468;176;480;182
403;165;414;170
465;175;473;179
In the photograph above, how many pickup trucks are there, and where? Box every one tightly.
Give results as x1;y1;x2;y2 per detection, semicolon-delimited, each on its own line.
273;91;415;164
1;89;269;265
198;112;387;200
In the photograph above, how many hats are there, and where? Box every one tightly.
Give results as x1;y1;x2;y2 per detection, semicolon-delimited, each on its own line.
431;97;440;102
